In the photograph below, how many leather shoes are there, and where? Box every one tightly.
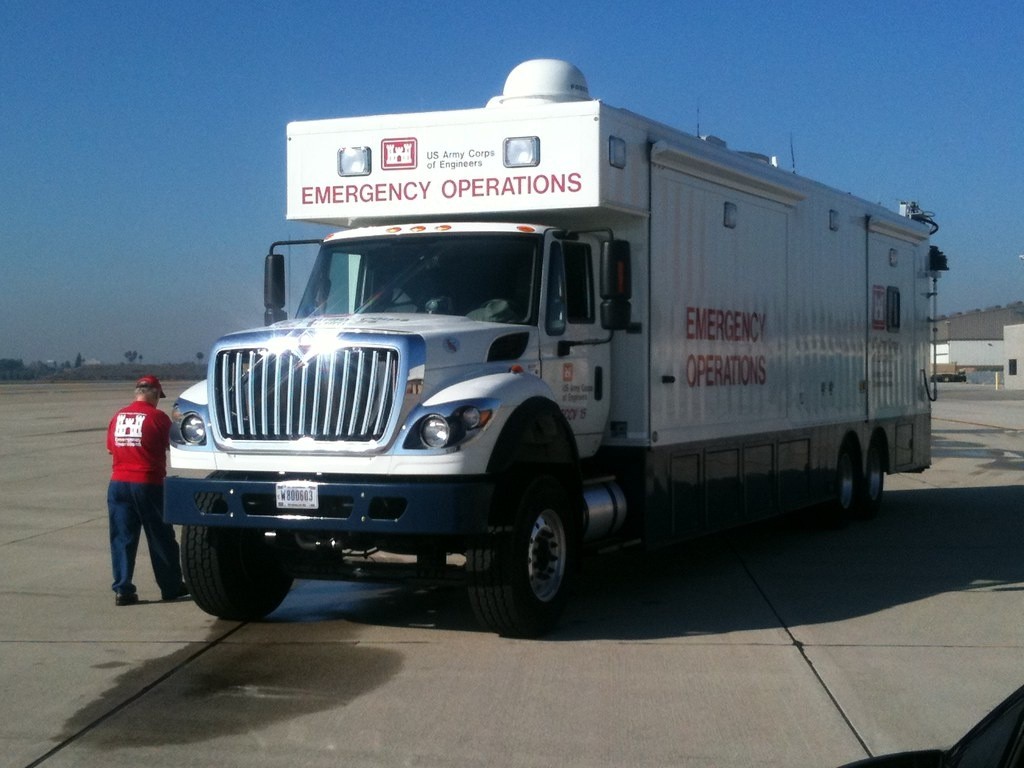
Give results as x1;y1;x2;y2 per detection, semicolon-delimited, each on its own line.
160;581;189;601
114;592;139;606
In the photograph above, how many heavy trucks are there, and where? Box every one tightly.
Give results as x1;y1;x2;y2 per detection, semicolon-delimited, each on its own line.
162;59;953;639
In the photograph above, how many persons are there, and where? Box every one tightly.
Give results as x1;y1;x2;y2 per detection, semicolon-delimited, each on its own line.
107;375;190;605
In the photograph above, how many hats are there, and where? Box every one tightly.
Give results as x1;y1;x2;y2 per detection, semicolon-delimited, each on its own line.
136;376;166;398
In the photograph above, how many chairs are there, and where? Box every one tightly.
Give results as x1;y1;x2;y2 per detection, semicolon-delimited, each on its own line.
416;256;468;313
